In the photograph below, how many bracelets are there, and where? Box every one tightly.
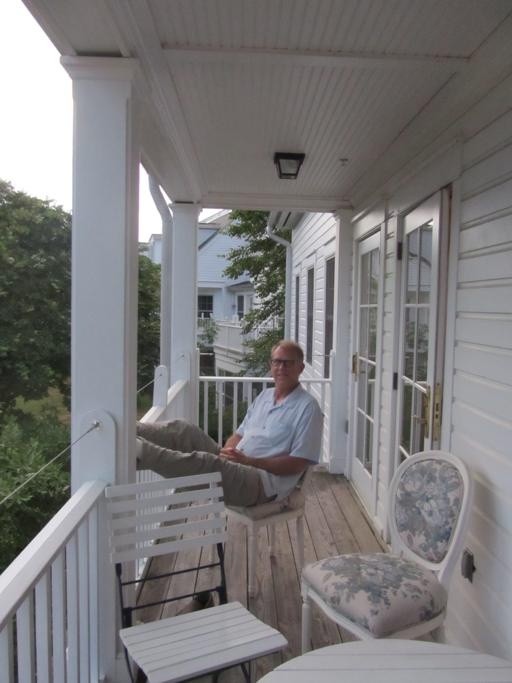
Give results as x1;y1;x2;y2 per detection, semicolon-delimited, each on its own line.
251;460;262;470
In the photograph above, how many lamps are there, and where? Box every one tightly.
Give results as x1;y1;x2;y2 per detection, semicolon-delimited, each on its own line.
272;150;306;180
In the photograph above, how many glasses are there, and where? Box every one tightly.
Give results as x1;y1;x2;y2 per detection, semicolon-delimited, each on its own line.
271;359;300;367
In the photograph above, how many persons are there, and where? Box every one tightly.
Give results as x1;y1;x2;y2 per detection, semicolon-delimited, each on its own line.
133;340;325;508
